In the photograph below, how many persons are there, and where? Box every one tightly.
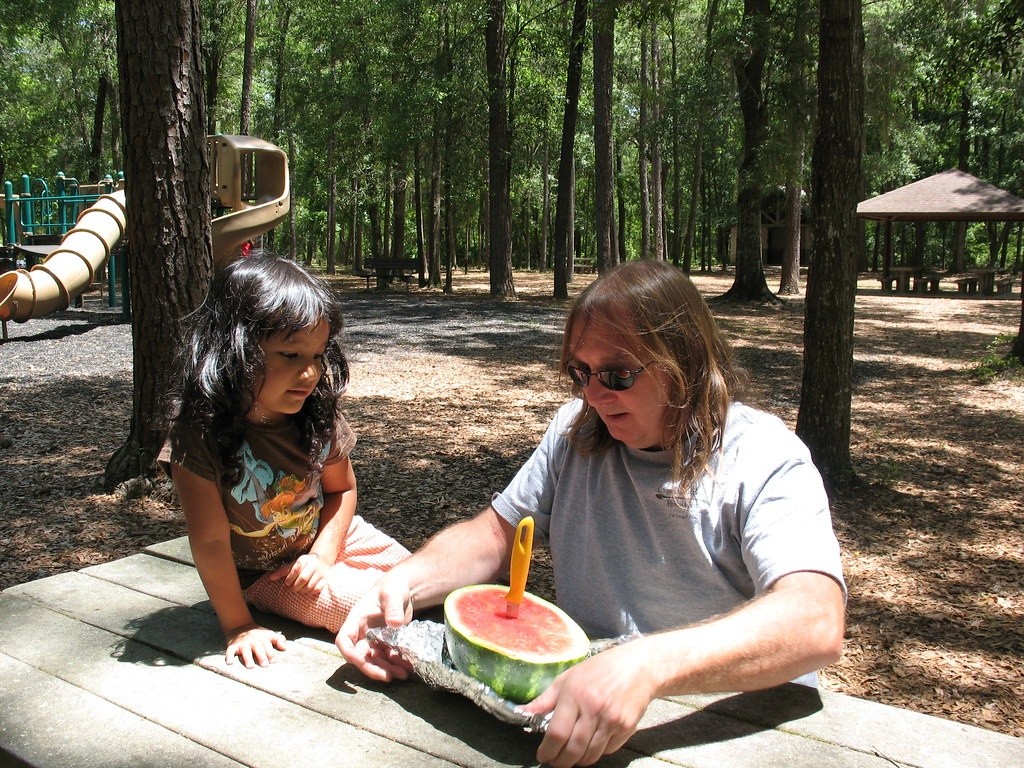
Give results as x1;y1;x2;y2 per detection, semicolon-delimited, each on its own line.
334;258;847;768
139;251;416;670
240;239;255;258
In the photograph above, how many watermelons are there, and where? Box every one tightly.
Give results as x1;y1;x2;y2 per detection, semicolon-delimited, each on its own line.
443;584;591;703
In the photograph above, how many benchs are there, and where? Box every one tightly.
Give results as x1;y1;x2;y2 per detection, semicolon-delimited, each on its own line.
877;274;912;292
915;275;943;293
993;278;1016;294
565;257;595;274
353;257;418;292
954;278;979;294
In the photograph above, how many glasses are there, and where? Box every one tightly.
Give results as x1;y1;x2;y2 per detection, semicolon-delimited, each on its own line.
563;359;657;391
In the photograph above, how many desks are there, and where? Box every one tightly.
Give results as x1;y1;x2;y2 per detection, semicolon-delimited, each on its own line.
968;268;1008;294
1;533;1024;767
889;265;930;294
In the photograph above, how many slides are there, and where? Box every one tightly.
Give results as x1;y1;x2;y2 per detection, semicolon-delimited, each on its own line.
0;148;291;321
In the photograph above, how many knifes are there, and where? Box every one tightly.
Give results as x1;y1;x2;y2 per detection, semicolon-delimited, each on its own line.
503;516;534;618
